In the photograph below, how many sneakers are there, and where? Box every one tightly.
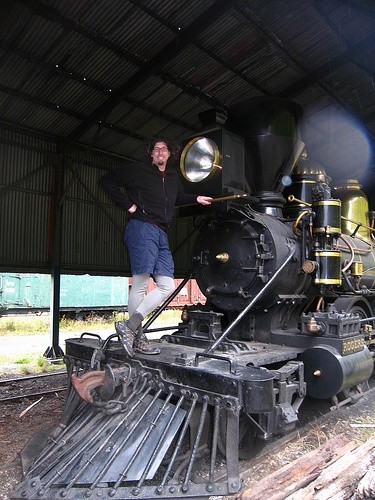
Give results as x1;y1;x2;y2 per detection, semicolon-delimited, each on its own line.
133;334;160;354
115;318;136;359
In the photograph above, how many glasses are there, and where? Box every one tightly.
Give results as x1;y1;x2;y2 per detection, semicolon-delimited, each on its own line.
153;146;168;152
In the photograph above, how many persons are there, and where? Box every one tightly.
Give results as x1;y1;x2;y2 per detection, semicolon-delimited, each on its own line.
99;134;214;358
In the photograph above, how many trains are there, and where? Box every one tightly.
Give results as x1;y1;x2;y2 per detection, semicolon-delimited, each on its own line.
8;92;375;500
